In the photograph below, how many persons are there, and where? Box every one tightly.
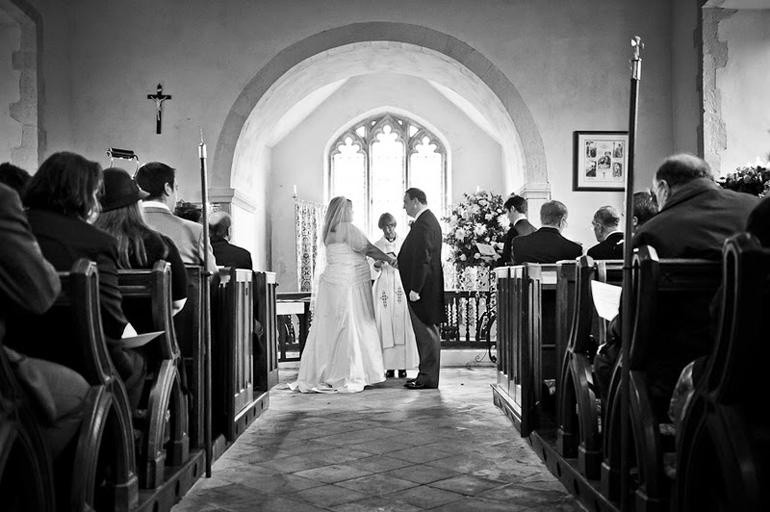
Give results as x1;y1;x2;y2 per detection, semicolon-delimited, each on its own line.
494;197;538;266
134;163;217;280
1;163;32;205
368;213;420;378
2;185;90;512
171;200;200;221
667;196;770;421
92;168;187;360
21;153;147;415
209;212;263;347
587;205;624;259
586;140;624;180
594;153;762;396
286;196;396;393
389;188;447;389
512;200;582;264
612;192;658;258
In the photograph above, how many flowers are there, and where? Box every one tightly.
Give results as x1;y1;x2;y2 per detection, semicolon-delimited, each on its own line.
439;190;511;296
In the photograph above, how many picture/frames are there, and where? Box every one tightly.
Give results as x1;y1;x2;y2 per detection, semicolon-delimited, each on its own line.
573;129;632;193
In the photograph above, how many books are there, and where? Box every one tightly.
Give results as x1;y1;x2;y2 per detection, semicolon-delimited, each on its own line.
475;242;495;255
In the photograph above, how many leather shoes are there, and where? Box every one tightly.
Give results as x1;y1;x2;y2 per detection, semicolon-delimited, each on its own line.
405;380;438;389
384;368;396;378
397;370;406;378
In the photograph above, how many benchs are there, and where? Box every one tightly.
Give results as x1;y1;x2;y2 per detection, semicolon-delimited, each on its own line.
1;342;57;512
255;272;287;396
115;259;192;490
183;262;225;454
489;257;595;480
597;242;723;511
218;266;256;417
674;231;765;511
553;254;632;503
56;257;139;511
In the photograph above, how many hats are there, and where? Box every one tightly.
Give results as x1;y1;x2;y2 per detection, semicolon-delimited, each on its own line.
96;167;151;212
